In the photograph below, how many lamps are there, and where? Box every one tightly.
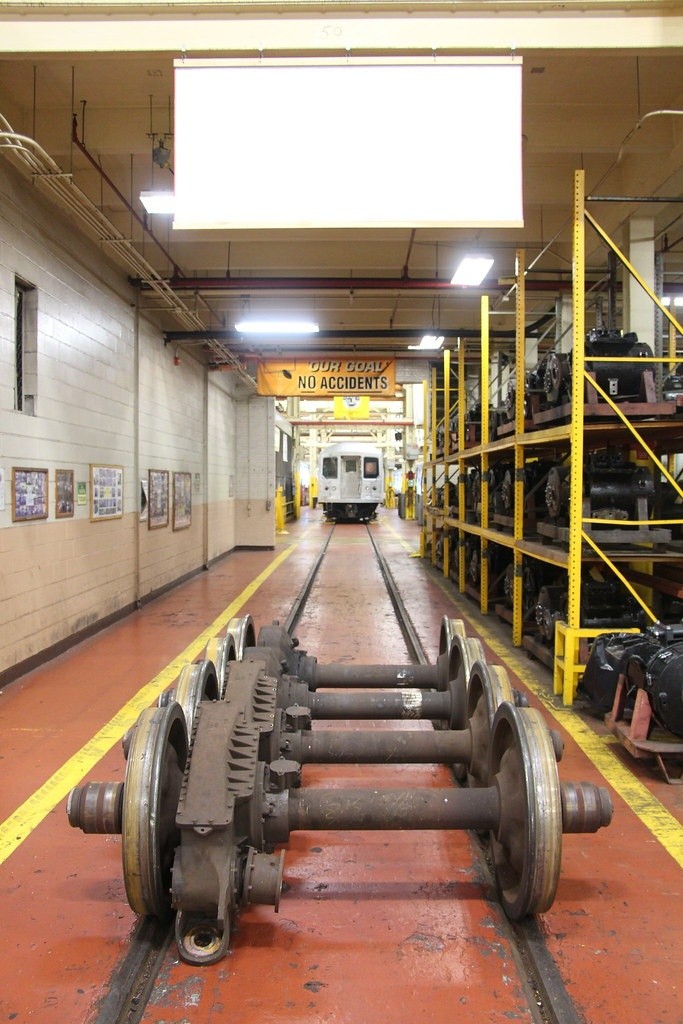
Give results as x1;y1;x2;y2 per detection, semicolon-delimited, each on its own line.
139;190;174;214
451;253;495;286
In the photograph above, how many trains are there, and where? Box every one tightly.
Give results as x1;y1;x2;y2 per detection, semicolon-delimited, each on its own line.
319;441;384;525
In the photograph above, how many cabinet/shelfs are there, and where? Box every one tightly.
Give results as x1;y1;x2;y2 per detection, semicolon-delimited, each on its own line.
423;168;683;706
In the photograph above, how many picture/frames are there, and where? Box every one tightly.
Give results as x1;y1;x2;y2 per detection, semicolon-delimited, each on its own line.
173;473;192;531
55;469;75;517
149;469;169;528
89;463;123;522
12;467;49;522
